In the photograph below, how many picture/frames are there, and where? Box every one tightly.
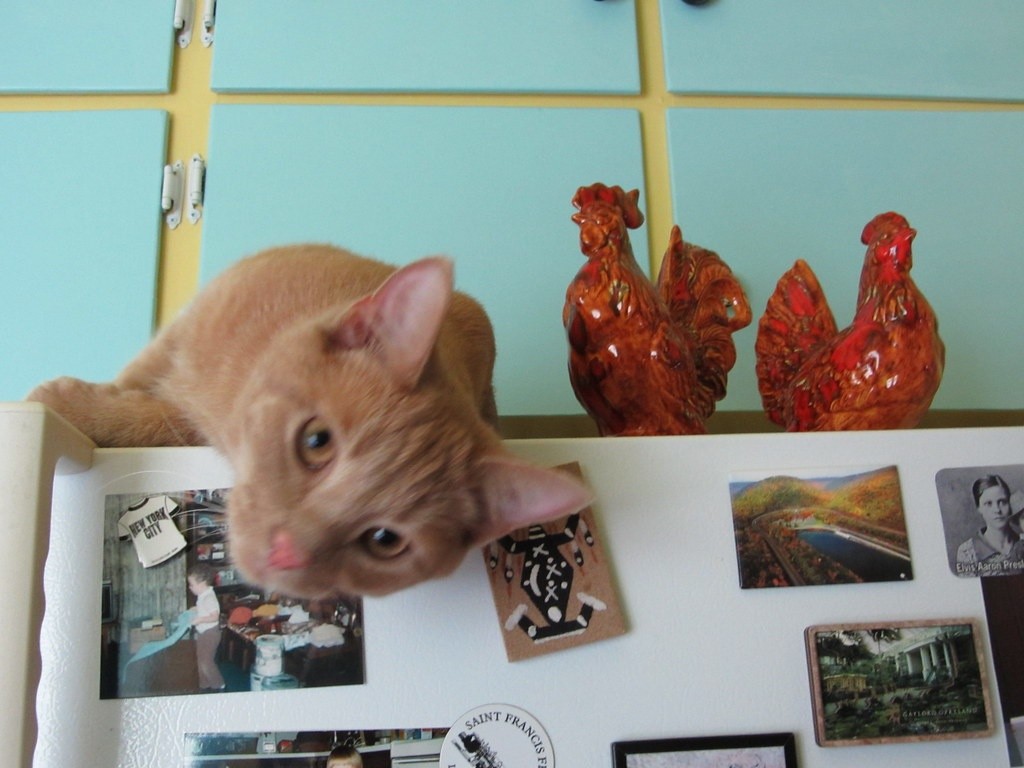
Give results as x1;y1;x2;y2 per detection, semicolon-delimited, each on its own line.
805;617;995;747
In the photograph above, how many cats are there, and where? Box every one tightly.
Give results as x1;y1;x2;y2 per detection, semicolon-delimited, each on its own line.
25;243;594;603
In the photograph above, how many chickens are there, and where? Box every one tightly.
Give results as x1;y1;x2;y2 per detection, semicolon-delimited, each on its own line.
563;181;945;436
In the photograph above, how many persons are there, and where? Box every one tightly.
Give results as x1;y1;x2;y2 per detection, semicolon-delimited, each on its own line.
957;474;1024;575
180;562;228;691
326;746;363;768
